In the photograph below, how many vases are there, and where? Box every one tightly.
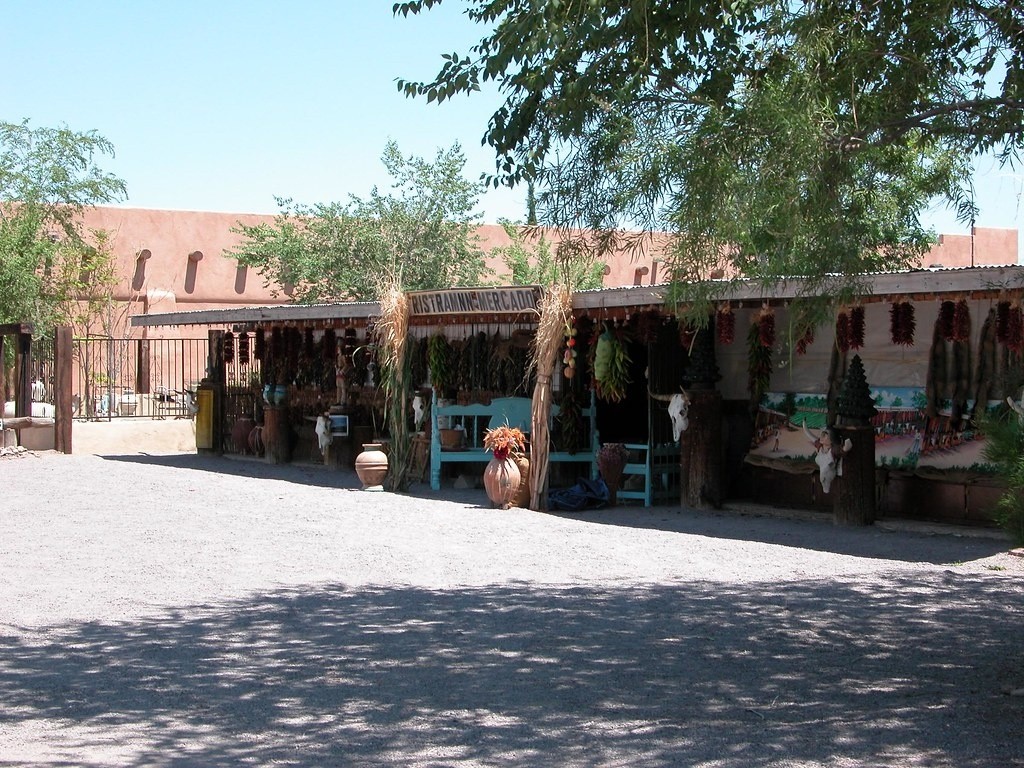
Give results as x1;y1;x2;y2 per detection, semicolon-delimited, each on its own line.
440;429;462;447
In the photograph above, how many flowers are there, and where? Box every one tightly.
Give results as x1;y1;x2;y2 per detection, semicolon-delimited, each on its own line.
597;442;630;505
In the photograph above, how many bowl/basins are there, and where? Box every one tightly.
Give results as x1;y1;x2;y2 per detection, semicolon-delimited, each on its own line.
439;428;464;448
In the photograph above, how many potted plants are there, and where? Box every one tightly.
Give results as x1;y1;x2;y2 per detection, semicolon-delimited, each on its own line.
482;415;527;460
263;359;295;406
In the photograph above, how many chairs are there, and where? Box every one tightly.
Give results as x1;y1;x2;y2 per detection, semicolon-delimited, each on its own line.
152;386;182;420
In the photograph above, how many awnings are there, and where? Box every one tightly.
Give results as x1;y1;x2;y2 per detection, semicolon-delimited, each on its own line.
129;302;382;328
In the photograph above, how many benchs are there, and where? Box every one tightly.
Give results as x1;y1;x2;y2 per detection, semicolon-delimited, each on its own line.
601;405;682;508
428;385;598;490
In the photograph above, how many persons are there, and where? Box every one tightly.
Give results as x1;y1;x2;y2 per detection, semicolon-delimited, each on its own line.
335;345;347;406
